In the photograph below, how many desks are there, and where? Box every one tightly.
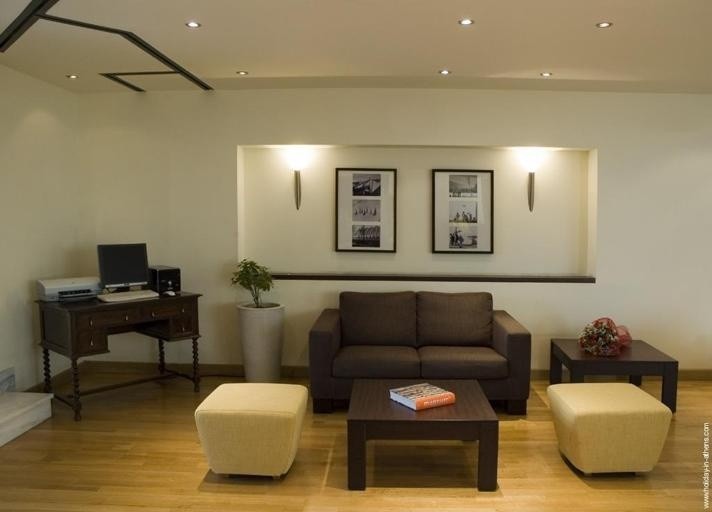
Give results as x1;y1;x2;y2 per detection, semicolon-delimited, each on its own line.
36;291;201;421
549;337;678;415
0;390;57;450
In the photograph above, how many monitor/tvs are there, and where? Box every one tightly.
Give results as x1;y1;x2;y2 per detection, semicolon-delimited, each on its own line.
98;244;149;293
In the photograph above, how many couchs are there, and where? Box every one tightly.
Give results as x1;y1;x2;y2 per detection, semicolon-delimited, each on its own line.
307;290;532;418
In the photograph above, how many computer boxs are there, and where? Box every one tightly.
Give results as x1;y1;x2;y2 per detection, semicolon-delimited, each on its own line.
142;265;181;294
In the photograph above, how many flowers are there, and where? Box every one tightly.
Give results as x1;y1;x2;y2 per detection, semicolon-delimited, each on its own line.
578;317;632;359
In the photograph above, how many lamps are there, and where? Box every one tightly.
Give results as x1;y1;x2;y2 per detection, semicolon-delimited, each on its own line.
510;146;549;212
277;145;317;208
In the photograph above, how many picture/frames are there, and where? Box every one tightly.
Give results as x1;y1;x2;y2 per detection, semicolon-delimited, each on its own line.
430;167;495;256
334;167;398;254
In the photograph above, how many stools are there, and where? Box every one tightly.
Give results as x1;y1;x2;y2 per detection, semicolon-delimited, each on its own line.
195;381;309;480
545;380;673;476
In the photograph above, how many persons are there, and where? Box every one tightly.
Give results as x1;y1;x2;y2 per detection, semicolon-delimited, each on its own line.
449;210;465;249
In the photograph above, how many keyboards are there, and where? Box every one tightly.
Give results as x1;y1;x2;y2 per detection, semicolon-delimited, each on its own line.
97;290;159;303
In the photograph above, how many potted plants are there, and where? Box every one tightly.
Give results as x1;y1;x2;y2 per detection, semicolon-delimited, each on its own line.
230;257;287;382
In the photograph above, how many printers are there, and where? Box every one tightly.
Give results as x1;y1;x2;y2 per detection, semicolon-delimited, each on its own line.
36;277;102;302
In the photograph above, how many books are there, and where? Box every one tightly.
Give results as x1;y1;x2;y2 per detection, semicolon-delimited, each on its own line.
390;381;455;412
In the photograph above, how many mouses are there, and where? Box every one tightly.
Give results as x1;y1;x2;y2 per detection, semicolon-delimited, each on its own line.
163;291;176;297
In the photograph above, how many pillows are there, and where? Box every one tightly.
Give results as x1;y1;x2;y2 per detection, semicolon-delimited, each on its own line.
340;289;417;346
417;290;493;345
